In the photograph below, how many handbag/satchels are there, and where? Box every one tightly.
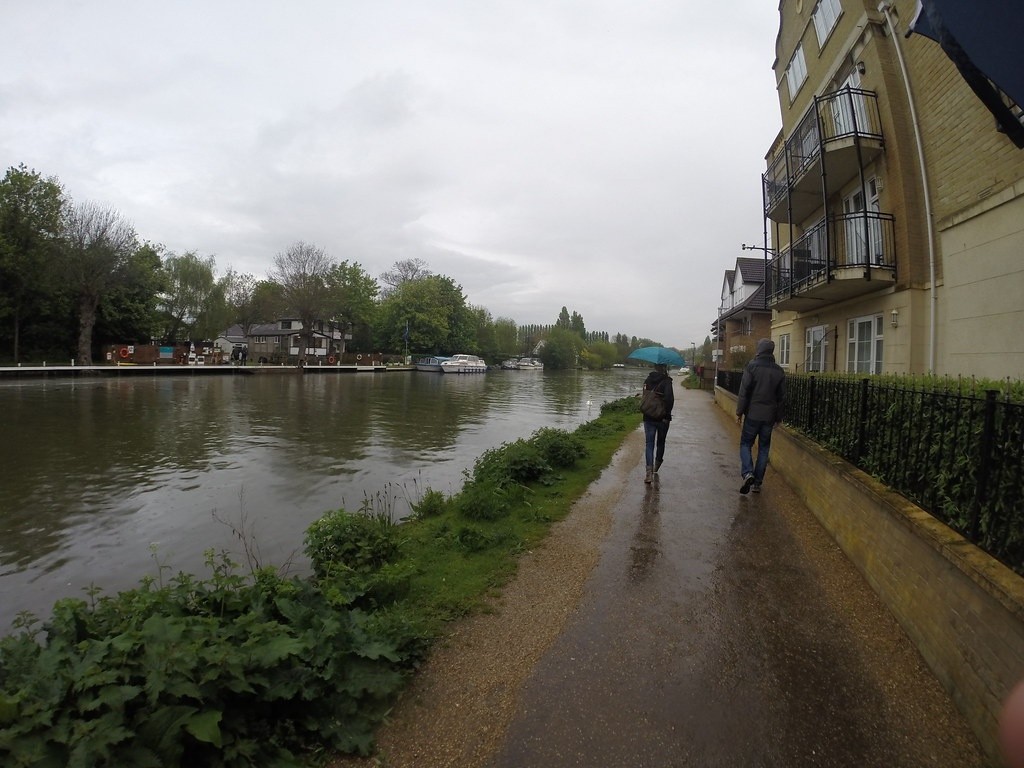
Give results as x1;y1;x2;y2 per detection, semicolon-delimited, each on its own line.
640;376;670;418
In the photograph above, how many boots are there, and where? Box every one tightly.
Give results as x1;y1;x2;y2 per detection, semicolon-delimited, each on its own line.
654;458;664;472
642;465;654;482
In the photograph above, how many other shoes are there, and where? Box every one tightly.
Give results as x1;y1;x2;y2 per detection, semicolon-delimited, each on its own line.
740;472;755;495
751;485;760;493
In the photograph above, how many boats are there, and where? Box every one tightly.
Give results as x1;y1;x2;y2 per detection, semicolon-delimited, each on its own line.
516;358;546;372
414;357;452;371
678;367;690;376
441;354;488;373
501;359;520;370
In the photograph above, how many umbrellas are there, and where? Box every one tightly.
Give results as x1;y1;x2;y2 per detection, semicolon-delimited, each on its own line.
628;346;685;366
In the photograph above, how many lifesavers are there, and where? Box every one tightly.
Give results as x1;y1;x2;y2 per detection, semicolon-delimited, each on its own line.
357;354;362;360
120;348;128;358
329;357;334;363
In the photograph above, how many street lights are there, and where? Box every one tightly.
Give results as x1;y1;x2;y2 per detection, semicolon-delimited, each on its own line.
691;342;695;371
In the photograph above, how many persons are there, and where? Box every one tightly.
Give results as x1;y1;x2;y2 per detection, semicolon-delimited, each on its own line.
735;338;786;494
641;363;674;482
240;345;248;366
231;346;240;360
